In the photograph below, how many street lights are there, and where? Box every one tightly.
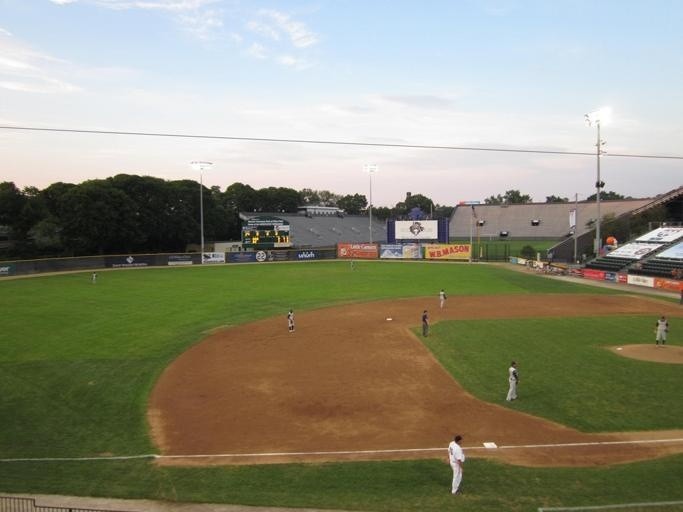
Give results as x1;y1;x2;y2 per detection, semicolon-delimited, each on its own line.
361;164;380;243
188;161;215;266
582;111;608;257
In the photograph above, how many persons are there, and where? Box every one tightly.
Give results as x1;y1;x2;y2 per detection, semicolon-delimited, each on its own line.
671;267;681;279
91;272;98;284
349;259;354;271
654;316;668;348
506;361;519;401
421;309;430;338
448;435;465;495
287;309;296;333
577;252;587;265
438;289;448;309
